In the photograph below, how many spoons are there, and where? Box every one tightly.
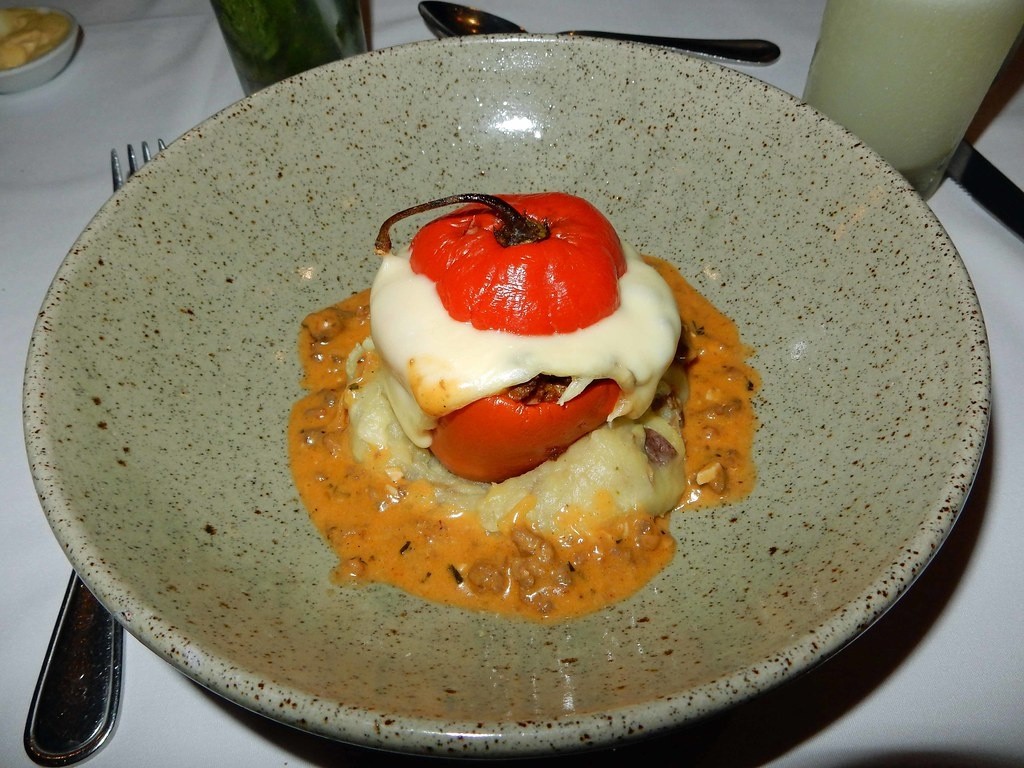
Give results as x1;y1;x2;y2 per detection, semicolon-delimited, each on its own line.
416;0;782;69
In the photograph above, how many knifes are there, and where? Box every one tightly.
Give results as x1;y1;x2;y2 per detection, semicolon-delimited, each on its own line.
945;137;1024;244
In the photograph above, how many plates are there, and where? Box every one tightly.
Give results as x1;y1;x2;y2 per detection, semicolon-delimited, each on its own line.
22;33;992;757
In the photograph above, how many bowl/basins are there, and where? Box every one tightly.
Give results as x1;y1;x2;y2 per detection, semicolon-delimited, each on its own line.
0;5;79;95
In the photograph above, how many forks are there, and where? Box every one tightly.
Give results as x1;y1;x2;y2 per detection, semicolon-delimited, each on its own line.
21;137;168;768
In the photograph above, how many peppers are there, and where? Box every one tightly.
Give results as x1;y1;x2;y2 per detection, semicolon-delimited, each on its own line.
368;191;625;484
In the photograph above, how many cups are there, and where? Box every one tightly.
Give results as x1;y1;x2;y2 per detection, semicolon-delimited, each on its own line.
208;0;372;99
800;0;1024;203
